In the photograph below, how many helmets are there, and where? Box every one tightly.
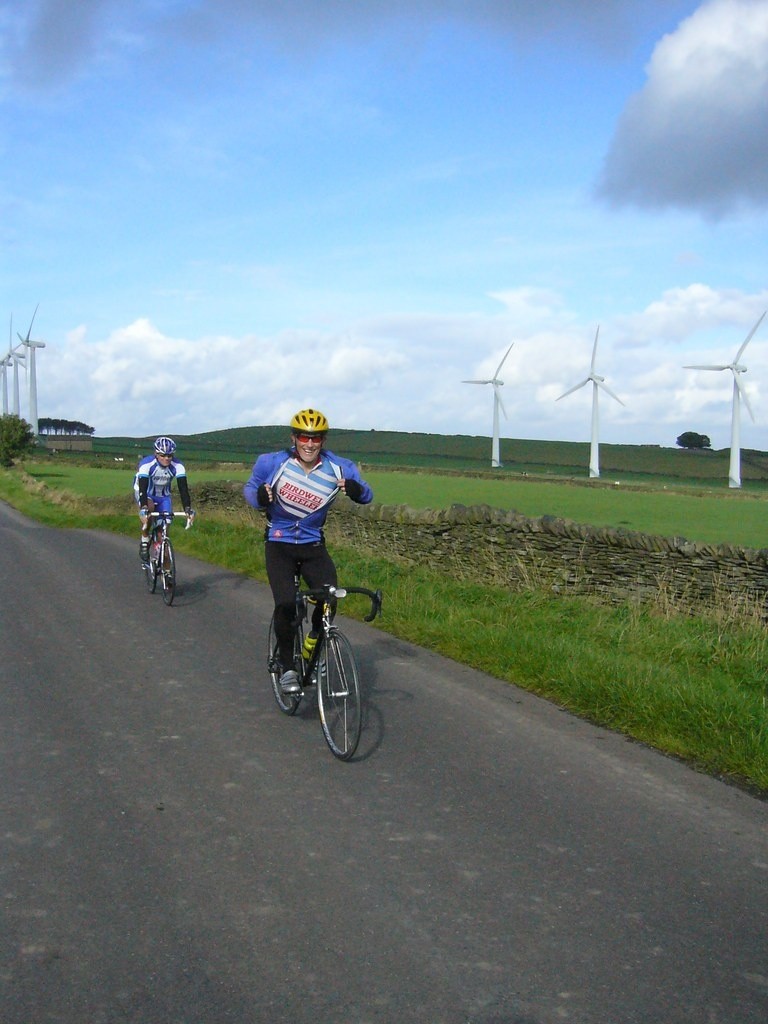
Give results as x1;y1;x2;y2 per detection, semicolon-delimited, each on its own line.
290;409;329;436
154;437;177;454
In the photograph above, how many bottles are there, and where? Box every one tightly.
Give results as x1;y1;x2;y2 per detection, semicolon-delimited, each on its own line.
301;631;318;660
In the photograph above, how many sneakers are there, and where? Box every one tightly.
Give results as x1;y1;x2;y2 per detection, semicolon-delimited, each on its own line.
279;670;301;694
139;541;151;560
164;572;173;591
313;658;326;677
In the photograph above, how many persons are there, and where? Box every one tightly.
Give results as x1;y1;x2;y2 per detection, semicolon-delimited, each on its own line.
133;437;195;587
244;410;374;694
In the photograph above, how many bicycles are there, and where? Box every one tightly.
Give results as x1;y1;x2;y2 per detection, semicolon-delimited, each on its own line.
268;558;382;760
141;500;197;604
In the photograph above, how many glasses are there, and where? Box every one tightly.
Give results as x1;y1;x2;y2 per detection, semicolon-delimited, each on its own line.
158;453;171;458
293;432;322;443
